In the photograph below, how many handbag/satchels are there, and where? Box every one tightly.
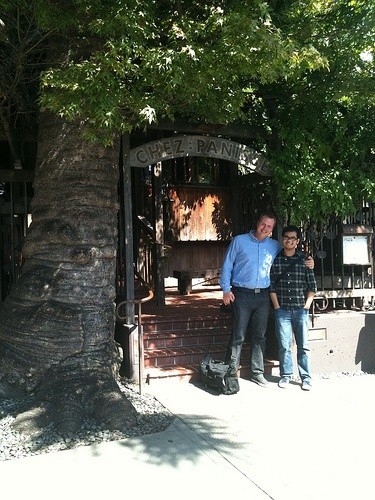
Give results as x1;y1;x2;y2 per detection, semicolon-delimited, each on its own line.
198;359;239;394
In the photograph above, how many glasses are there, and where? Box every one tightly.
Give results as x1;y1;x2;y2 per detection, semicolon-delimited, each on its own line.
283;236;299;241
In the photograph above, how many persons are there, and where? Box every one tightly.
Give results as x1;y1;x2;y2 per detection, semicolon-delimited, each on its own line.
221;212;314;386
269;225;317;391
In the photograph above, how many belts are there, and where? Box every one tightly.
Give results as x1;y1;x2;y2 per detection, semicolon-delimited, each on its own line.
235;286;269;293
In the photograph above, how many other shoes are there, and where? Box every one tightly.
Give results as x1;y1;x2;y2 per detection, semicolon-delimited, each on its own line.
251;374;268;386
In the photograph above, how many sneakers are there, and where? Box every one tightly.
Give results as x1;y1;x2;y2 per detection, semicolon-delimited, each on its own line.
301;378;312;391
277;376;290;388
